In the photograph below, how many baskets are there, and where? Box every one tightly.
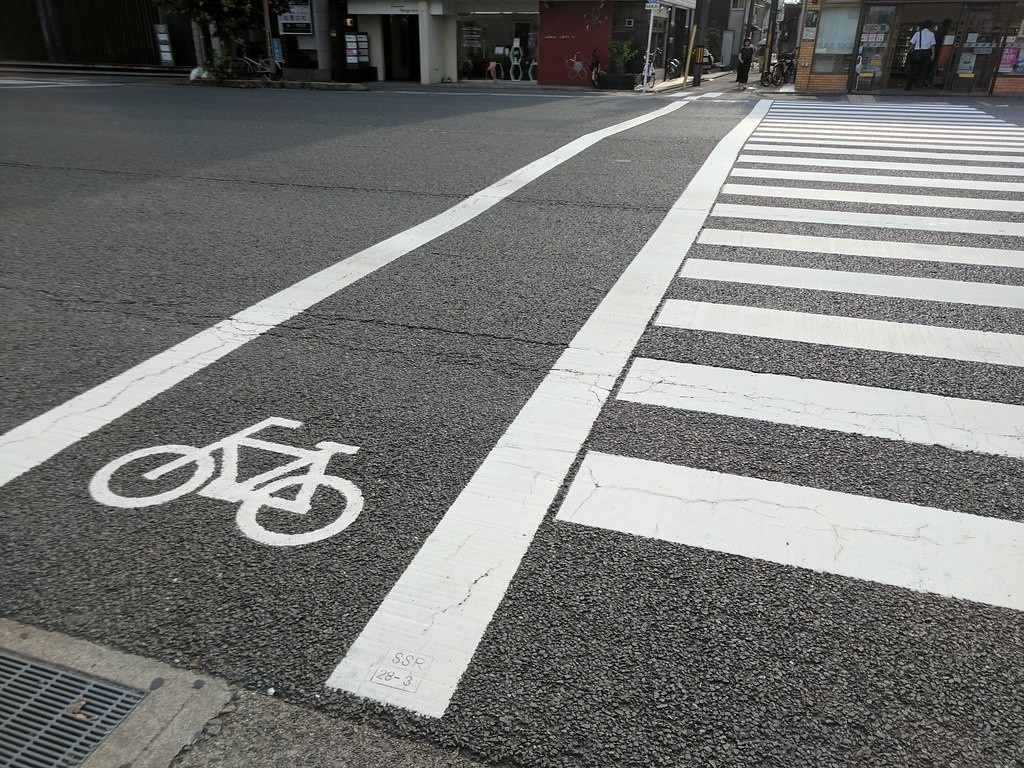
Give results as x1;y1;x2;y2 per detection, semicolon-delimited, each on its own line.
643;55;655;62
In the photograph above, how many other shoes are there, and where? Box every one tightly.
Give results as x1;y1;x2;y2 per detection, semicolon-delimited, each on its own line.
904;88;911;91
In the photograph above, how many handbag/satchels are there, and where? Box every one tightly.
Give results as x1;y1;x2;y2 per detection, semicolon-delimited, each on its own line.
907;50;926;66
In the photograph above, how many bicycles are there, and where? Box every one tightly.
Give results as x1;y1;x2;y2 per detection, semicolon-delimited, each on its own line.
760;46;800;87
230;41;283;82
589;48;607;89
640;47;662;88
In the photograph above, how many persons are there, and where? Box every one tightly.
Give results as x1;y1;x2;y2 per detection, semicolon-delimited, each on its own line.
904;20;936;91
736;39;754;91
936;18;953;57
